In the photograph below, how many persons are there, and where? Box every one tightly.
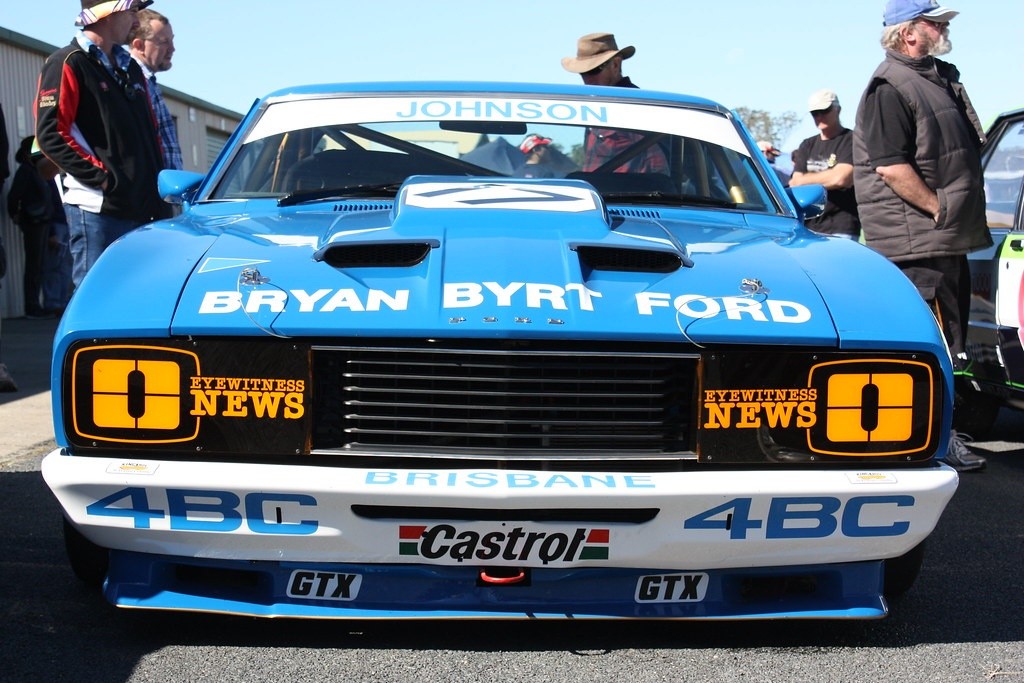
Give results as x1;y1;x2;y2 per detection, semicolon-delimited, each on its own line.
0;0;185;392
520;33;673;197
755;0;994;471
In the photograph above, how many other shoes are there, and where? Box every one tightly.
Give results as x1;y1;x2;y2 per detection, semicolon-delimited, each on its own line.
0;363;19;392
26;309;47;319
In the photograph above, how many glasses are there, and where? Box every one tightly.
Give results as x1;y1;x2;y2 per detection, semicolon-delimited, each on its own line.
915;19;940;27
588;58;614;73
114;67;137;101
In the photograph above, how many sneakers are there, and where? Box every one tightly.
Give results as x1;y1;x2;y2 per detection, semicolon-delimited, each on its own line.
943;429;988;471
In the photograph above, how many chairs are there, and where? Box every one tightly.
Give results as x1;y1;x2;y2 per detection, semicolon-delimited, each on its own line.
566;172;680;207
280;149;428;193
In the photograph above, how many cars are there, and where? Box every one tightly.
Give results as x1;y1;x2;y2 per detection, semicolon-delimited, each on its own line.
952;103;1024;442
41;83;959;622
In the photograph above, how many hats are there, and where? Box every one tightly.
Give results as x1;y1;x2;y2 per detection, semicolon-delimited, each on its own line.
882;0;960;26
757;141;781;156
16;135;35;163
75;0;154;26
560;32;636;73
519;134;552;154
808;90;838;111
